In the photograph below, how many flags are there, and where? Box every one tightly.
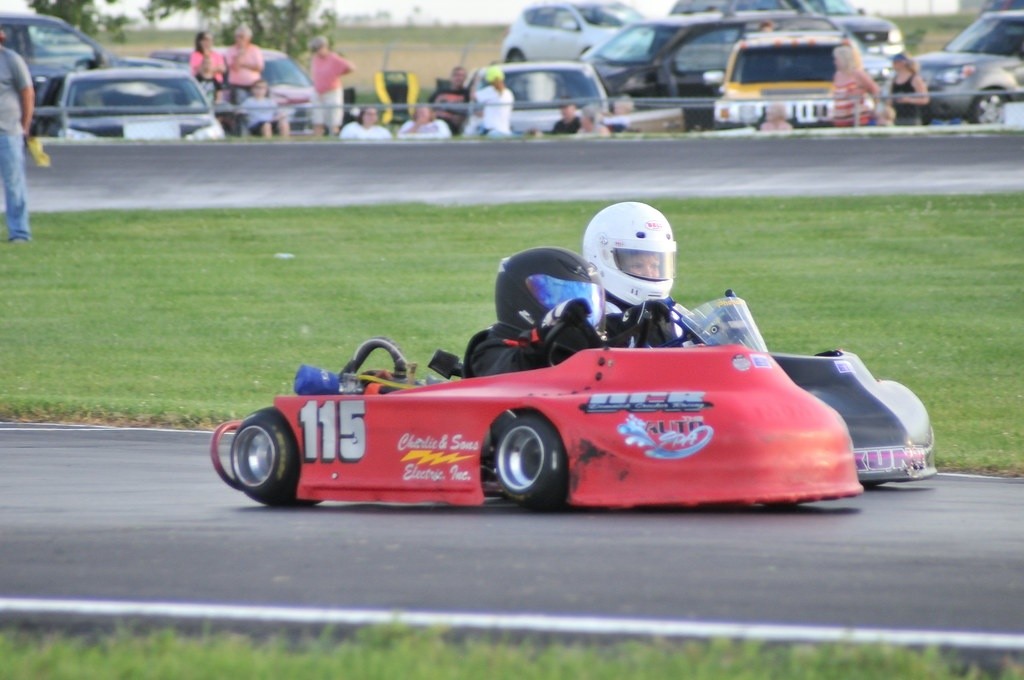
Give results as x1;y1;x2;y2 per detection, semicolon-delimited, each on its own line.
26;136;50;168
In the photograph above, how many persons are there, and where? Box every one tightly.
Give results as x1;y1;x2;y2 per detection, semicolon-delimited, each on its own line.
759;21;773;33
551;97;583;134
338;108;392;140
397;103;451;138
428;67;469;135
759;103;792;131
526;128;542;137
237;79;290;138
581;201;750;348
576;105;610;135
226;23;264;106
599;99;643;133
464;247;672;384
832;45;881;126
879;50;930;126
190;31;225;125
308;36;353;136
0;30;36;245
470;66;514;137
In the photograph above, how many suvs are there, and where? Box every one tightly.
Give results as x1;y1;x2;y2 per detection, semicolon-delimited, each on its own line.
704;31;864;130
575;10;891;131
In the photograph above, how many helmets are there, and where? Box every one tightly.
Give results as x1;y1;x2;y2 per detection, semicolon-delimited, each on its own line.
581;200;676;301
494;245;606;335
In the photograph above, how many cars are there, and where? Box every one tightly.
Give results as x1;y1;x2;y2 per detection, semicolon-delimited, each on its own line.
47;67;225;140
499;1;658;73
150;45;320;121
669;0;904;59
909;9;1024;124
466;59;613;133
0;13;177;135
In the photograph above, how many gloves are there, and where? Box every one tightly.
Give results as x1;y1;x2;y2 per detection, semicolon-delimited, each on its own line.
621;300;669;344
537;297;591;334
